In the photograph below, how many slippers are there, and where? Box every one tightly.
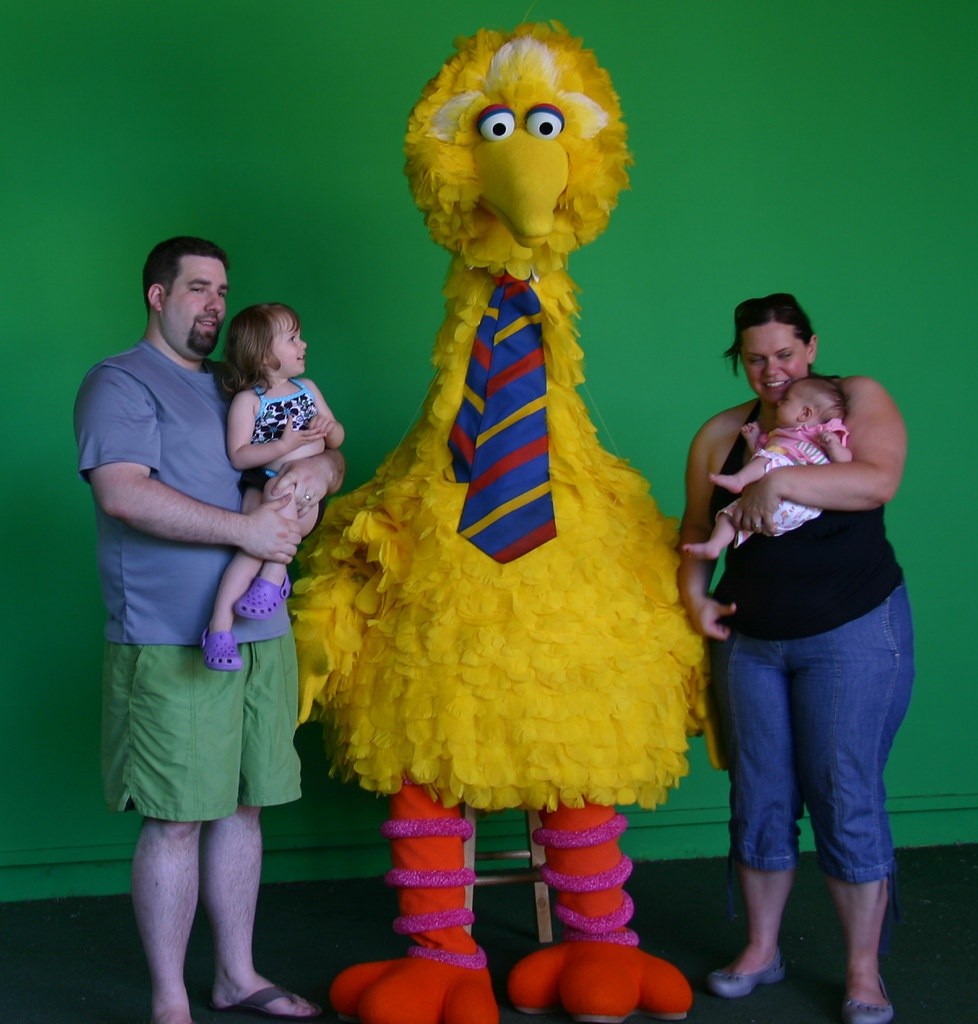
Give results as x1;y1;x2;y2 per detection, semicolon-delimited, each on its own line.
207;984;323;1019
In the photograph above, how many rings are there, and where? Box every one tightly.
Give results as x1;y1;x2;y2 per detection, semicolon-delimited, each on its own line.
752;524;760;529
304;495;311;501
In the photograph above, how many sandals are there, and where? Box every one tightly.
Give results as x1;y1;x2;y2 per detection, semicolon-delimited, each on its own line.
842;974;894;1024
234;571;291;619
705;945;786;999
200;627;243;671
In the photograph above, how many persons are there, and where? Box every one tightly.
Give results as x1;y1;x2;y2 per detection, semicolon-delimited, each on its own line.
201;302;345;669
676;293;914;1024
74;235;345;1024
682;377;853;559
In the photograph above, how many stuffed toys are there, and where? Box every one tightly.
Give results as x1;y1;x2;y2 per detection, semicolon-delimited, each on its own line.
289;23;723;1024
